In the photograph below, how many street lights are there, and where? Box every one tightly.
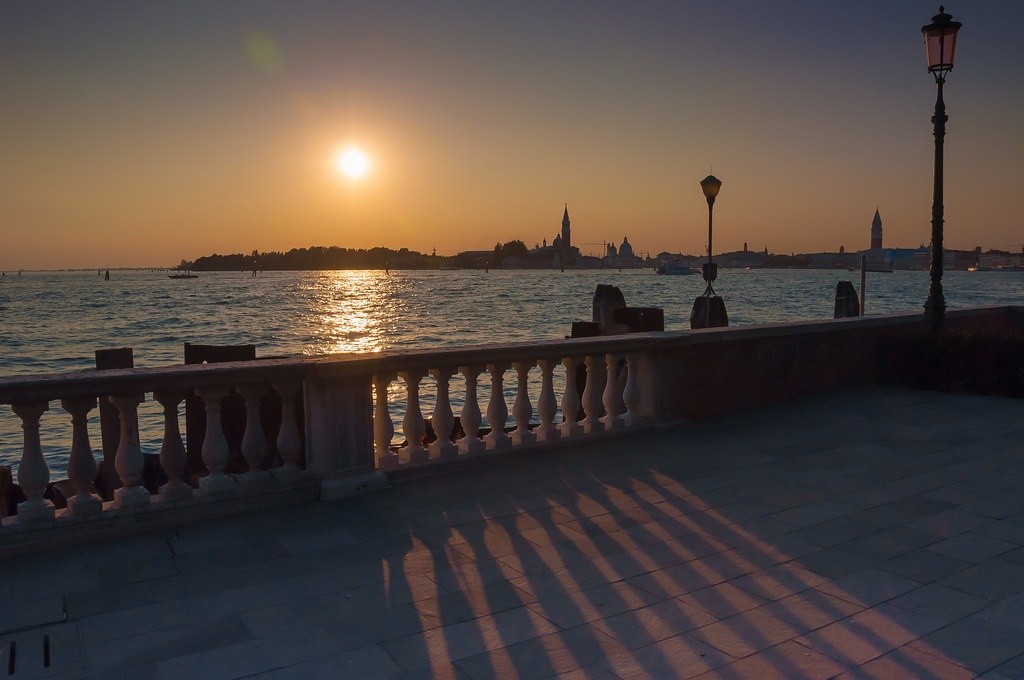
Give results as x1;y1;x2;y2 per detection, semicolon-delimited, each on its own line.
691;167;728;330
919;5;964;313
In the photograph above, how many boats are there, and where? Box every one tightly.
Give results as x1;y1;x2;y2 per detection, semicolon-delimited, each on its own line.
168;268;199;279
